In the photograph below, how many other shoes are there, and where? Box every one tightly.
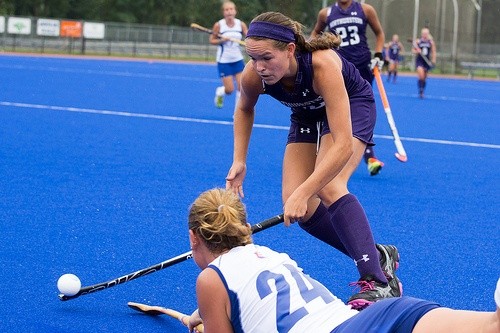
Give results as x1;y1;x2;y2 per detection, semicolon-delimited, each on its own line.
214;87;223;107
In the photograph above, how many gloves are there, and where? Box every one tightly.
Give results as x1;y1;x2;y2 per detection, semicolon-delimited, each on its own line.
368;52;384;72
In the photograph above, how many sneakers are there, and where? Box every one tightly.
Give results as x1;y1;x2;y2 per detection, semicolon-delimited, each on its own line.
346;280;396;311
375;243;403;298
367;157;384;176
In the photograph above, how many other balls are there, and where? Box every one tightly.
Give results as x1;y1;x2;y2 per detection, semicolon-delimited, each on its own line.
57;274;82;296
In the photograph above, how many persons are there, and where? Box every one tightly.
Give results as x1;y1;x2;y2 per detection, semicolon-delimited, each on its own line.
210;0;248;119
225;12;402;309
188;187;500;333
383;34;404;83
412;28;436;98
310;0;386;175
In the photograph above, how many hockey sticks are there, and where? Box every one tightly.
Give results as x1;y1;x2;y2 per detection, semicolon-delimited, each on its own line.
127;301;205;333
410;39;432;67
59;213;285;301
191;24;246;46
373;65;408;162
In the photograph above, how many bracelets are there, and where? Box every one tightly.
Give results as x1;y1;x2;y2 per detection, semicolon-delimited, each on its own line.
375;52;382;60
